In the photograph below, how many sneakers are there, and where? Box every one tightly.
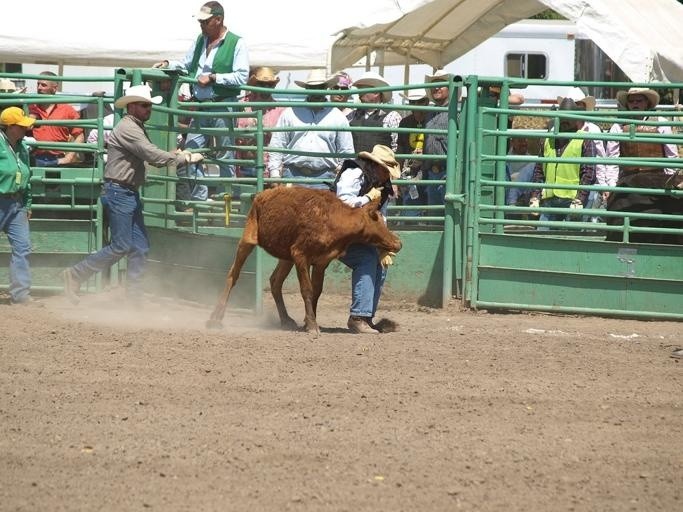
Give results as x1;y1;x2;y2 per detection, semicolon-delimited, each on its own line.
347;314;380;335
13;296;44;310
62;267;82;305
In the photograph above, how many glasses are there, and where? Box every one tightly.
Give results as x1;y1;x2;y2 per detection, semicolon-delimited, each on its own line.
198;19;219;26
131;101;153;110
626;96;647;103
331;85;349;91
259;82;275;87
309;84;326;89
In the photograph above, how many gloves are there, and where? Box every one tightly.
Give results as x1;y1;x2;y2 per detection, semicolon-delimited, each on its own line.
374;246;396;270
529;197;540;216
367;185;385;201
185;151;203;164
570;198;584;210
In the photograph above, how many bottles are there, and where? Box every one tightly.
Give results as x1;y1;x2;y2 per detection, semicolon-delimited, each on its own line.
406;175;419;200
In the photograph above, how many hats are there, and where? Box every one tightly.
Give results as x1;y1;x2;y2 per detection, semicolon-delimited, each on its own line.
191;0;224;21
0;106;36;128
351;70;393;103
247;66;281;86
113;85;164;108
399;88;429;101
423;70;462;103
294;66;340;89
557;87;597;112
358;143;401;179
551;97;587;111
0;78;28;95
615;86;660;112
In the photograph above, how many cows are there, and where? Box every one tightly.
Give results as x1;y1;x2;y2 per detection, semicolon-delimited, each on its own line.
205;185;403;341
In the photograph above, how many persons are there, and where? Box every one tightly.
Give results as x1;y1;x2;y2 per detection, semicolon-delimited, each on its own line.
147;1;464;231
27;71;120;196
0;107;45;308
477;82;682;244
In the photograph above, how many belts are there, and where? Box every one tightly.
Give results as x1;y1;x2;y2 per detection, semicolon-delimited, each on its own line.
192;95;231;103
